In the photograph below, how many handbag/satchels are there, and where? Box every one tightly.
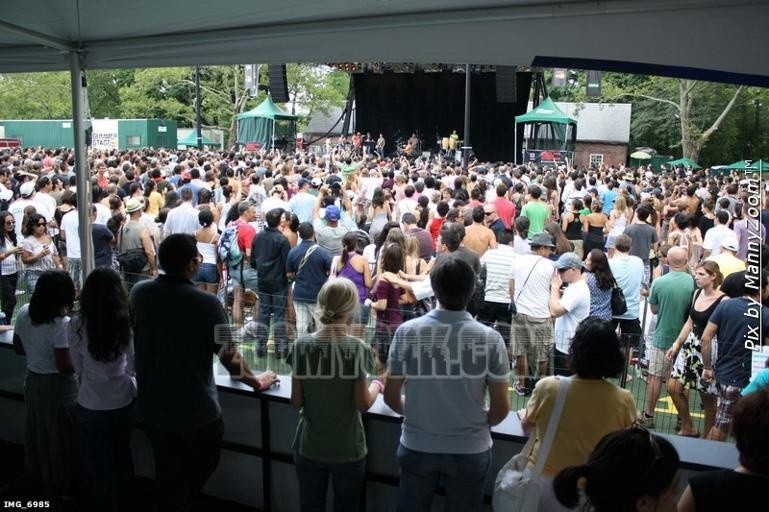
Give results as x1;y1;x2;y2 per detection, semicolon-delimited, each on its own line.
610;285;628;316
492;454;541;512
116;249;148;273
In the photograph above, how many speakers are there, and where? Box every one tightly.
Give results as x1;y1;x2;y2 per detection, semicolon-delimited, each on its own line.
494;63;515;104
268;63;289;103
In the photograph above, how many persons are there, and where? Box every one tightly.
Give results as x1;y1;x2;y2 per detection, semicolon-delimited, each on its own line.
0;130;769;512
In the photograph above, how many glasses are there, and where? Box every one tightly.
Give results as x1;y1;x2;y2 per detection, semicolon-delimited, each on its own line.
35;223;46;227
558;267;571;273
6;220;16;225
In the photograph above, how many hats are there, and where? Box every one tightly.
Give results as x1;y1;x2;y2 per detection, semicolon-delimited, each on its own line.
678;202;688;212
326;205;340;221
20;181;35;197
312;177;323;187
528;233;555;247
587;188;598;195
125;199;144;213
622;173;635;181
0;190;13;202
721;237;739;252
552;252;582;270
650;188;661;195
273;185;285;193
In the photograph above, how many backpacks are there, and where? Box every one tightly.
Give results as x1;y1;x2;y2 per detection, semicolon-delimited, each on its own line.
217;222;244;268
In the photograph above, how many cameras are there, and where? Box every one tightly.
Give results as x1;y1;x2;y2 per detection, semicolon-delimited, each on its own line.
328;180;341;196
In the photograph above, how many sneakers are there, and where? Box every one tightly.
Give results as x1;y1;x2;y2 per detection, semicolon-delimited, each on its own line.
513;381;525;395
637;410;654;428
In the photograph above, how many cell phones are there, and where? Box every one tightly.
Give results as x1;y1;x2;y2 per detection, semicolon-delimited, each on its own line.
43;244;48;249
